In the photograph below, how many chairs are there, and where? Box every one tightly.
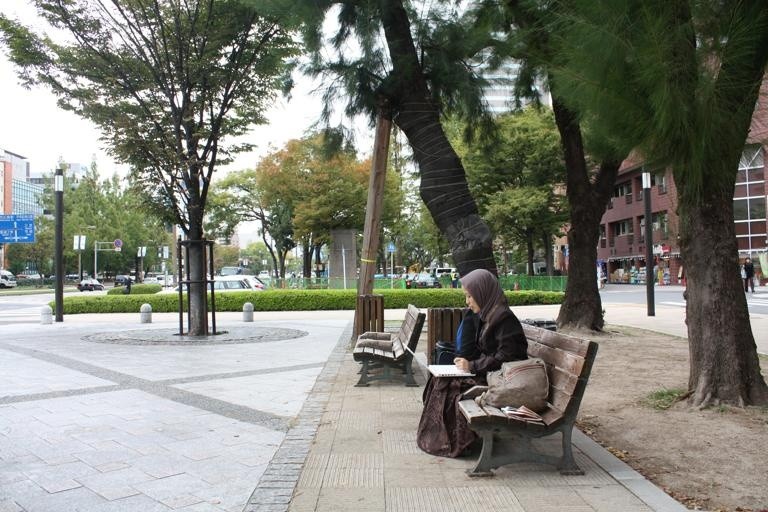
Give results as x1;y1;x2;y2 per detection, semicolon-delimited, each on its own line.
353;300;426;387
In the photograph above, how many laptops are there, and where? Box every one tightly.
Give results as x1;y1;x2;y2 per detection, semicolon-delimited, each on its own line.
402;342;477;377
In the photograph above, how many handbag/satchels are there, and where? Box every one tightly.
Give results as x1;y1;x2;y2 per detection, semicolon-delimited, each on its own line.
741;267;747;279
474;357;551;415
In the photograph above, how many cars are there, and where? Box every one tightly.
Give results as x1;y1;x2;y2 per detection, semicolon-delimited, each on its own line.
113;274;267;295
77;279;104;291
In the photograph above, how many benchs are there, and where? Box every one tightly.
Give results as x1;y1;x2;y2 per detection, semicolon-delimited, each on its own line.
458;321;599;477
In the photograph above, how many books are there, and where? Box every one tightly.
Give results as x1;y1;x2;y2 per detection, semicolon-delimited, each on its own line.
429;362;476;378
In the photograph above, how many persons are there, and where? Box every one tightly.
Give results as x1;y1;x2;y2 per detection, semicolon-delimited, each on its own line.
451;271;458;288
742;257;756;293
417;268;528;458
